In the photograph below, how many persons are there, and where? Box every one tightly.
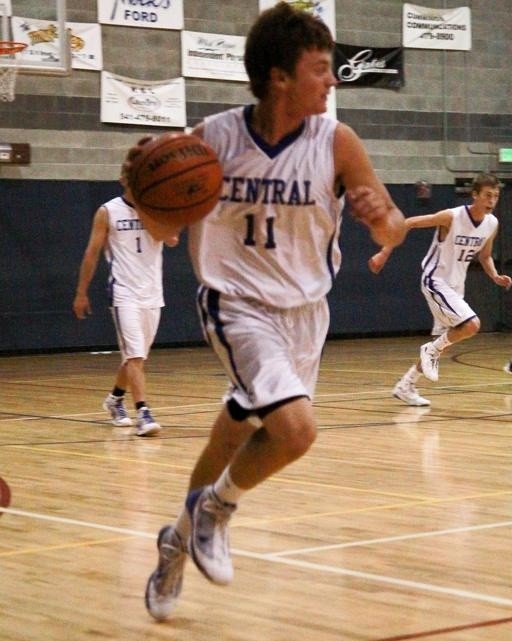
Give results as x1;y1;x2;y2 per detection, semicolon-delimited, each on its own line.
120;3;408;622
366;172;512;408
74;189;184;435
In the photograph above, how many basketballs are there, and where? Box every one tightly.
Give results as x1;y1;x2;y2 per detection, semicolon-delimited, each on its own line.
130;132;223;225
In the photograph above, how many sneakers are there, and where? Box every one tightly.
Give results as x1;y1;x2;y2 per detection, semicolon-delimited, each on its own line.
146;527;188;622
104;393;133;427
135;407;160;436
185;486;236;585
419;341;440;383
393;381;431;406
